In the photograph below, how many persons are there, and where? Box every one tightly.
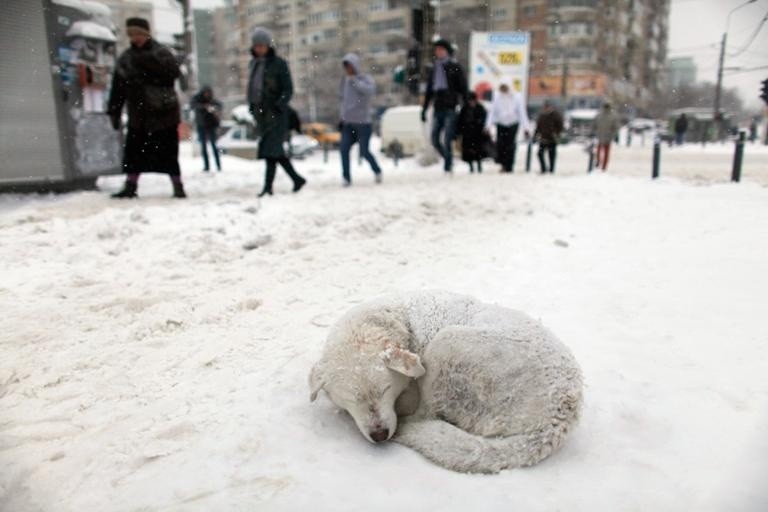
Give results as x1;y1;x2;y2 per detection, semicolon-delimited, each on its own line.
481;83;530;172
245;25;307;198
338;50;382;187
451;91;492;173
421;38;468;173
189;86;224;173
673;113;687;144
590;102;617;170
108;16;189;200
532;98;565;173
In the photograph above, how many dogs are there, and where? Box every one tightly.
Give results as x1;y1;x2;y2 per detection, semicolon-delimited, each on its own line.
308;286;587;477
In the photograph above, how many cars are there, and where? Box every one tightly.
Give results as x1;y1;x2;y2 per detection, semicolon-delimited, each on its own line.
562;104;737;146
217;117;345;158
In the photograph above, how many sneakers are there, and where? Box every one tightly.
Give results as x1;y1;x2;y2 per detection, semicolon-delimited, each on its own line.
173;183;186;198
374;169;382;184
258;185;272;197
341;178;350;188
110;182;138;197
293;178;305;193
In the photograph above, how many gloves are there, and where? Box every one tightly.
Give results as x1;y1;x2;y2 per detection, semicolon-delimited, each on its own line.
420;108;428;123
108;111;126;129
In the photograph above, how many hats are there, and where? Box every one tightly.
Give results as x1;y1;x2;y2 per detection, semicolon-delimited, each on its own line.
125;17;150;36
251;30;271;46
435;39;453;54
